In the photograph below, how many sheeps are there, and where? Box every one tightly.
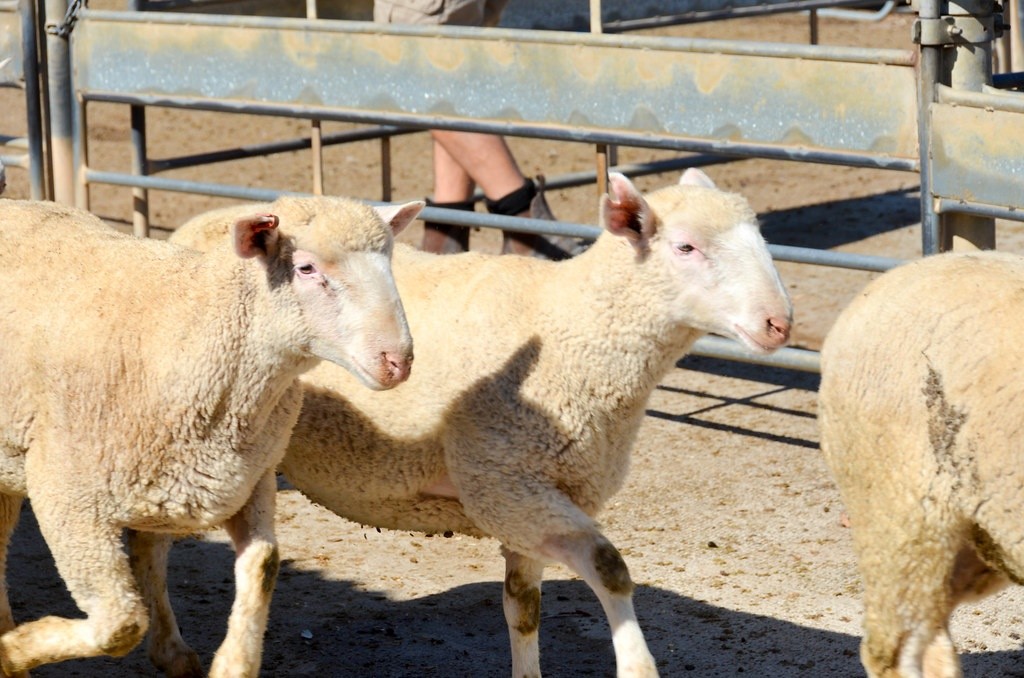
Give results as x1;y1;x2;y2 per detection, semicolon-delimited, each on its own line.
815;249;1024;678
1;198;424;678
122;166;795;678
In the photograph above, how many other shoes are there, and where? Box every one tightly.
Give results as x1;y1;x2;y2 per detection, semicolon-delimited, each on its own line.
420;217;471;253
495;175;584;261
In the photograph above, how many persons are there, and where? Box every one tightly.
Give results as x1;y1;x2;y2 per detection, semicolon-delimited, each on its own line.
373;0;586;262
